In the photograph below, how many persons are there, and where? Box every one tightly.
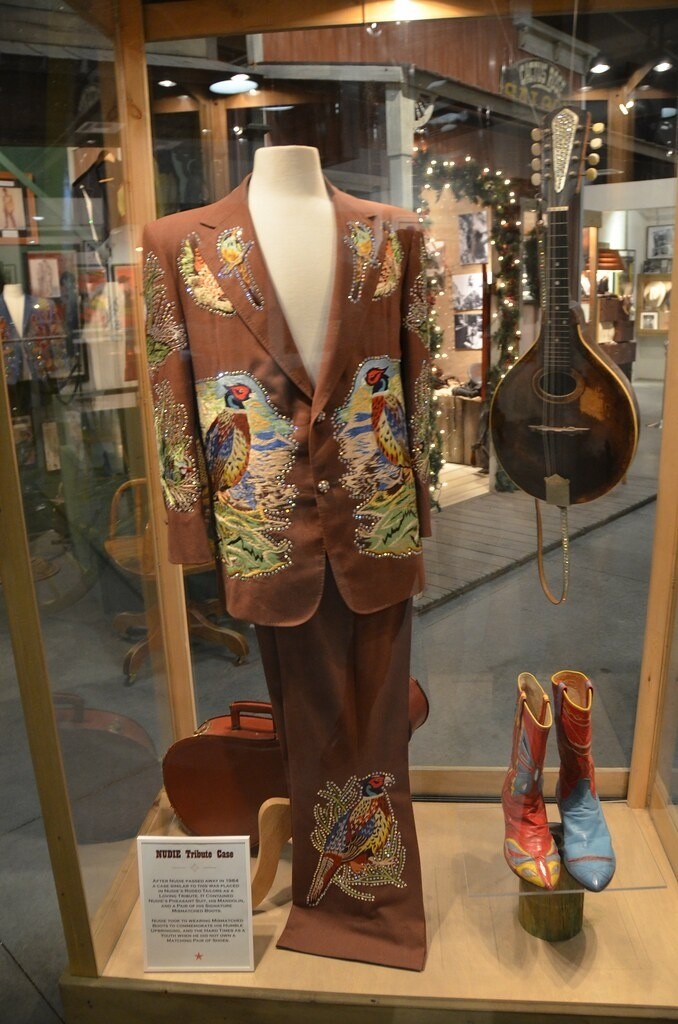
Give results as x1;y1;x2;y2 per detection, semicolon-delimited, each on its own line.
141;146;434;973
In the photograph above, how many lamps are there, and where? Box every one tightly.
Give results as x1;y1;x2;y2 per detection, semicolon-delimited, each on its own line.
588;51;612;74
653;55;674;73
208;68;261;101
156;72;180;89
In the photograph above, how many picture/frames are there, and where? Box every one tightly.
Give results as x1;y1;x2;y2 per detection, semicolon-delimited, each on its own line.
3;264;17;284
0;171;40;247
22;251;65;304
640;312;658;329
453;313;484;351
456;211;490;265
452;271;492;310
646;224;672;261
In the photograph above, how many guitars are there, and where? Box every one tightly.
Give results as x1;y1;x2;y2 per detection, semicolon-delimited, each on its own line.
489;104;641;508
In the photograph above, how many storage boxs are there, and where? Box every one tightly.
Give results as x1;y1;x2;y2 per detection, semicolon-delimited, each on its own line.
636;273;672;337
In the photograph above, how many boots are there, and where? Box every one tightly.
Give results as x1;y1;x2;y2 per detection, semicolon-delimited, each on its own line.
551;669;616;892
500;671;561;891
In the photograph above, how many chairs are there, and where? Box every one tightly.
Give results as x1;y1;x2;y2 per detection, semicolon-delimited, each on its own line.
104;477;250;684
